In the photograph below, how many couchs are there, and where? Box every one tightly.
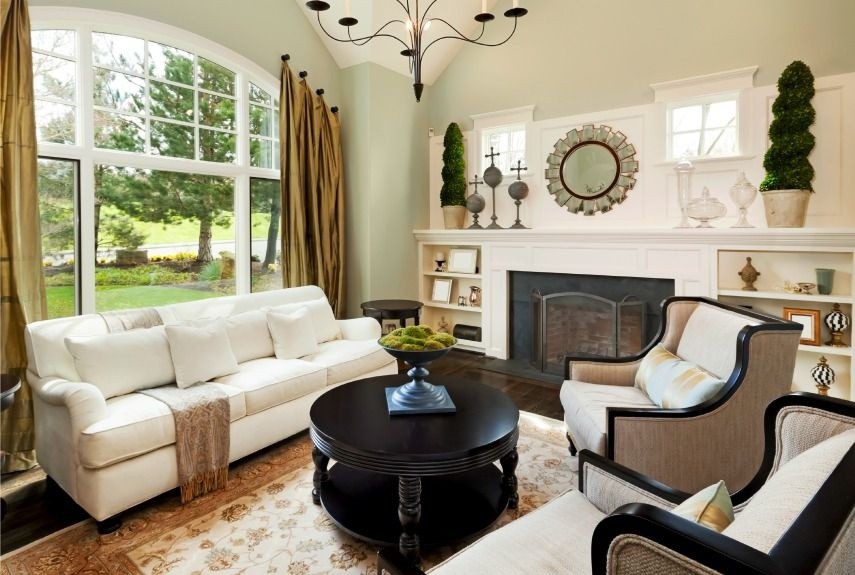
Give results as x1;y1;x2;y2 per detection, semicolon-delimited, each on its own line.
25;284;399;536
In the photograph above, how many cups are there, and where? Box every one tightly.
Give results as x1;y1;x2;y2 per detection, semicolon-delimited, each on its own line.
816;269;835;295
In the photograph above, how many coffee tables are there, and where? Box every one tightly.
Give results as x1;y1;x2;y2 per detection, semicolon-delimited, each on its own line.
308;374;519;566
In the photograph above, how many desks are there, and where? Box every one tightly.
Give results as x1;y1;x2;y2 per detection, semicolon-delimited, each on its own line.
0;373;22;521
359;299;424;328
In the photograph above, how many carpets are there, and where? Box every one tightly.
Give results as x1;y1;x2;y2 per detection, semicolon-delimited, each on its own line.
0;410;582;575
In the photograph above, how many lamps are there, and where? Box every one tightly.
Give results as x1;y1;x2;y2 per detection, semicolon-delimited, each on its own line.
304;0;529;105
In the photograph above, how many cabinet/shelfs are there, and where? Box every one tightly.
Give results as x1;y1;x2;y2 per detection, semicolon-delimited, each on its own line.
413;228;855;401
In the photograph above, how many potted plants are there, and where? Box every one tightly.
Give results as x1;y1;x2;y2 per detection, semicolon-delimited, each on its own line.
377;325;457;416
758;61;815;228
439;122;468;229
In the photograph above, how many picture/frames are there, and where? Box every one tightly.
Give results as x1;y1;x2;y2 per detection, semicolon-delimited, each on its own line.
783;308;820;346
447;249;478;274
431;279;452;304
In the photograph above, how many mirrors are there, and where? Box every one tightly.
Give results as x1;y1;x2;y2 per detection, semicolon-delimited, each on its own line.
543;123;639;217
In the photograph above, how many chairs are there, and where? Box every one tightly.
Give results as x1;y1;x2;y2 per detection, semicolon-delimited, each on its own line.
377;390;855;575
559;294;804;497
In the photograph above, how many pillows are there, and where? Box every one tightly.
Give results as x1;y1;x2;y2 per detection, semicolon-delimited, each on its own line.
165;316;239;389
269;295;341;344
192;307;275;362
634;342;727;409
668;479;736;533
62;322;182;399
265;306;318;360
